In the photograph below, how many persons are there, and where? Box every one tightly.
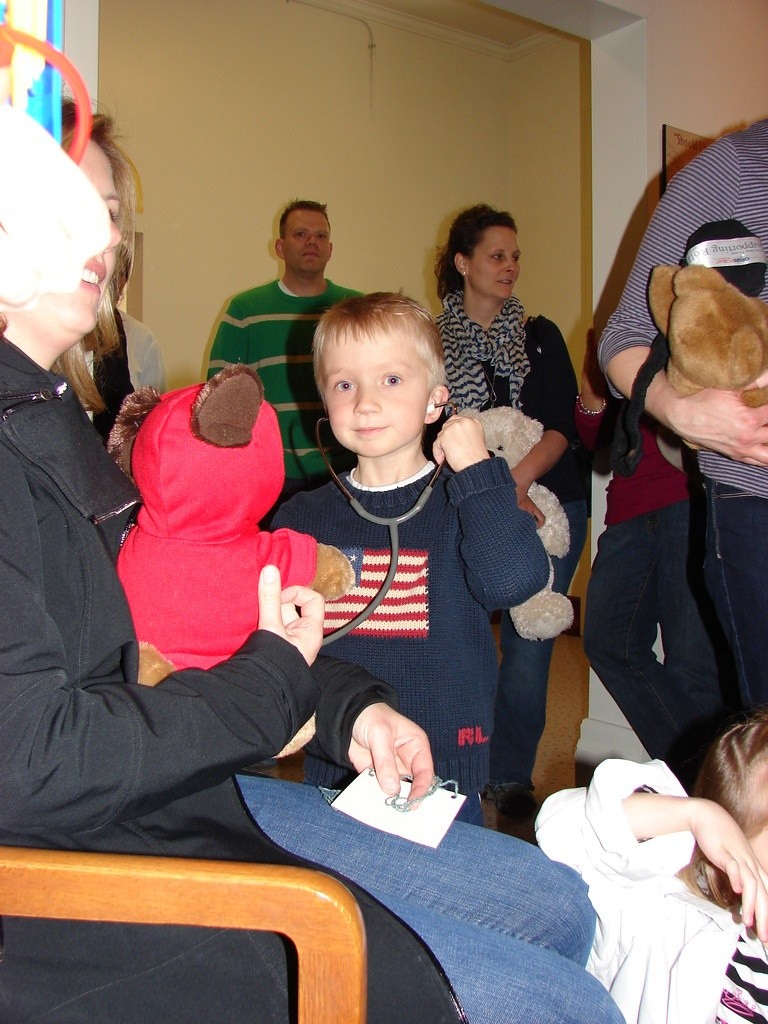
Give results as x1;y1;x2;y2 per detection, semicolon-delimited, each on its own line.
272;292;551;829
90;116;767;795
534;716;764;1023
0;98;626;1024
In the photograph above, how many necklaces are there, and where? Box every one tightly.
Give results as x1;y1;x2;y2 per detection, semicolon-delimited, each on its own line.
479;359;499;410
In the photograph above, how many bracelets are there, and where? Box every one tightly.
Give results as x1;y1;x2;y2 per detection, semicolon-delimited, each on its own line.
575;393;607;415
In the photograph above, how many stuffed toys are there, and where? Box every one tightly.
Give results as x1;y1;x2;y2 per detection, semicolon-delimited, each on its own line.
454;405;570;643
108;365;356;767
611;216;766;477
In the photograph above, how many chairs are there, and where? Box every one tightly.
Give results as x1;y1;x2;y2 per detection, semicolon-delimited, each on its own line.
1;847;365;1024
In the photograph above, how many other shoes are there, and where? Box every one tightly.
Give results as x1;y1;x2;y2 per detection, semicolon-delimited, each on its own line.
480;784;539;817
